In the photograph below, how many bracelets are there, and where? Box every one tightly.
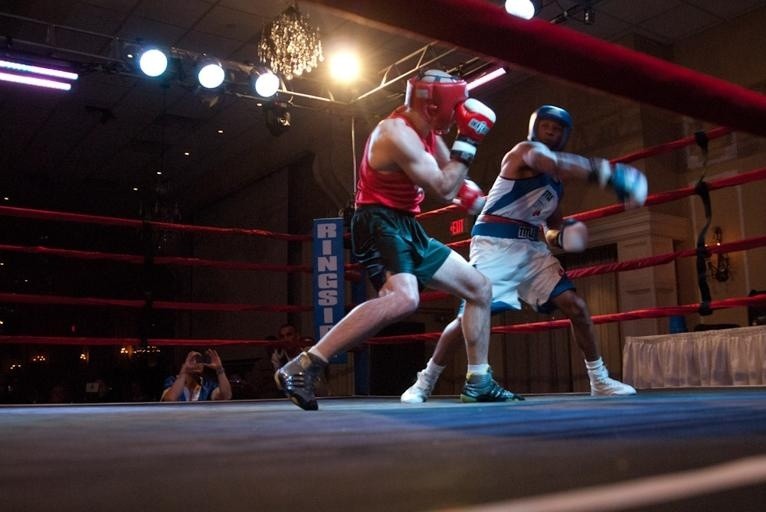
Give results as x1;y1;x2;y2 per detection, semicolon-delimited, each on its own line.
215;365;225;374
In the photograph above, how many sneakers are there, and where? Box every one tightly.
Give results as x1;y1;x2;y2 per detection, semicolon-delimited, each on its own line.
401;369;437;405
590;379;636;399
274;350;319;410
461;369;526;404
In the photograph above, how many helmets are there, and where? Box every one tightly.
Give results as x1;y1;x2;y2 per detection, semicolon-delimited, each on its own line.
526;105;572;152
405;65;468;135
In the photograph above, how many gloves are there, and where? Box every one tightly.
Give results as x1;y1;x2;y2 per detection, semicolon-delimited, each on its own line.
451;178;489;215
545;220;590;254
450;98;498;168
589;155;649;208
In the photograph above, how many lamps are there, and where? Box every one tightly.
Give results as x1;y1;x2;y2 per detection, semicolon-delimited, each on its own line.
121;43;280;98
466;66;508;90
256;0;325;81
505;0;545;20
0;52;79;91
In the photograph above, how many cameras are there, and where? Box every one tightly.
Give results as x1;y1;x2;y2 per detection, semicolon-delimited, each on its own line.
195;353;213;363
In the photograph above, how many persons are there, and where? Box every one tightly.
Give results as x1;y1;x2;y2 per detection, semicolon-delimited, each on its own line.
160;347;234;401
271;323;312;373
274;69;525;410
49;383;69;402
398;103;650;405
